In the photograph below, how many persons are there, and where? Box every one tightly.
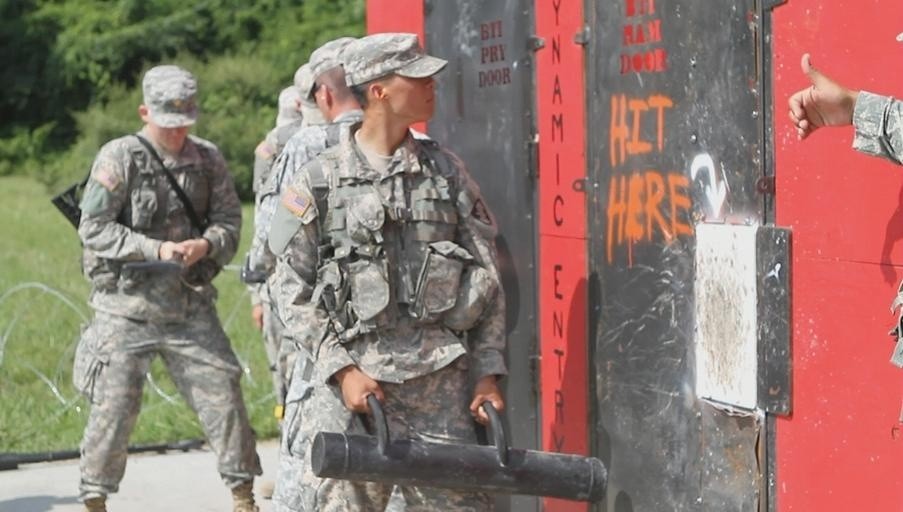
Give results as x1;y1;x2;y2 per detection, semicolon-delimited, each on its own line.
271;33;506;511
788;32;903;367
254;36;408;511
73;65;263;512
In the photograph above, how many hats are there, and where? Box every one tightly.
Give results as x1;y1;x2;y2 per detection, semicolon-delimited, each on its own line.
142;64;199;129
276;35;356;123
341;31;450;88
